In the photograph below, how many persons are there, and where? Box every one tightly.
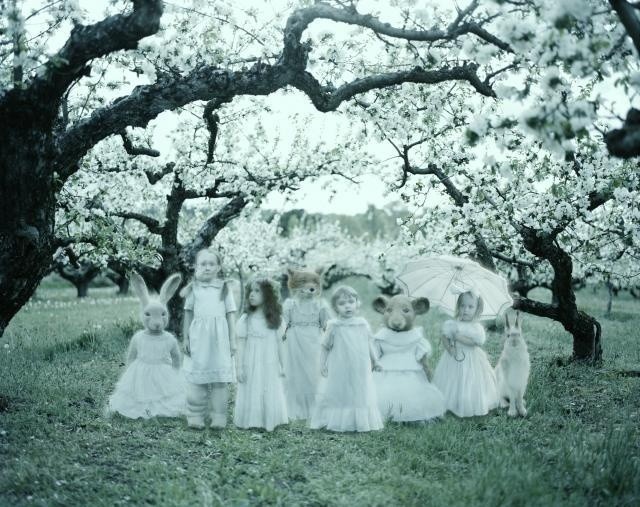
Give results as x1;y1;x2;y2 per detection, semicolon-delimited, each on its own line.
232;272;290;432
307;285;384;434
179;250;233;430
430;287;500;420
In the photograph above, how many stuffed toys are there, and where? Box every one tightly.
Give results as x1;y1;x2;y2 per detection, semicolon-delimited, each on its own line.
370;294;449;424
277;265;335;422
108;267;191;420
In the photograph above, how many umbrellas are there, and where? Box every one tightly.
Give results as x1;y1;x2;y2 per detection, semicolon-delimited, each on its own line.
394;251;514;321
